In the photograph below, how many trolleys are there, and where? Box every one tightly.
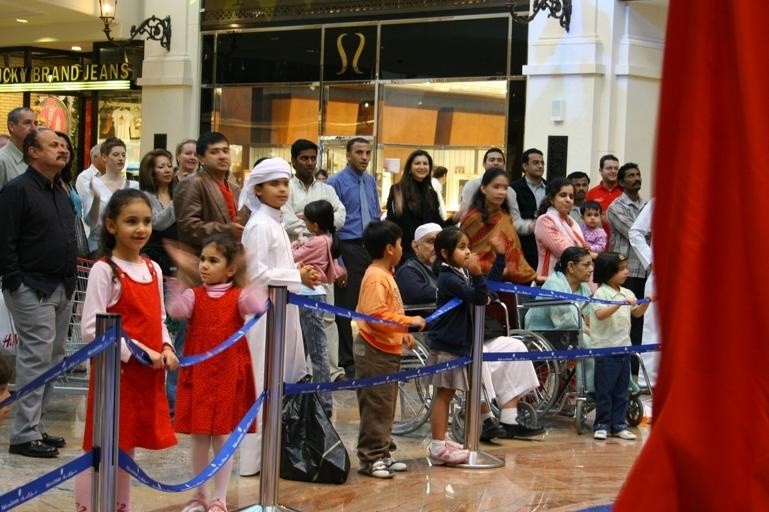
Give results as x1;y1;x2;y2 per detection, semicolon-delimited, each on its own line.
0;255;178;398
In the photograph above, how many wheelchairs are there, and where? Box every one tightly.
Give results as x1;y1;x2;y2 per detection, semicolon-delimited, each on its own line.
389;298;544;444
483;285;651;435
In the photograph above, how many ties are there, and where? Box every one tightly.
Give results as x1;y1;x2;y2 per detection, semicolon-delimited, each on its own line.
358;176;372;234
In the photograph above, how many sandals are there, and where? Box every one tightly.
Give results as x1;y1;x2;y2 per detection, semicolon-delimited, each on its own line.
207;499;228;512
180;495;207;512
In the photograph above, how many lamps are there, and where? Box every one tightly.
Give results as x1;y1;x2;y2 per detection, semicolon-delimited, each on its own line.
95;1;172;50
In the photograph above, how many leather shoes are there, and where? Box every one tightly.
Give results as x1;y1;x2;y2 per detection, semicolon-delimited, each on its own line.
36;435;65;447
497;420;546;438
479;417;506;441
9;440;59;457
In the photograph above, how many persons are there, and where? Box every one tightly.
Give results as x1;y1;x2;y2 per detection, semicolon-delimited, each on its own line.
431;167;449;220
386;150;465;241
0;340;18;425
0;128;79;458
55;131;89;266
174;133;246;245
587;251;658;441
523;247;642;401
351;221;425;479
0;106;37;191
239;156;307;478
281;139;351;381
462;147;538;237
316;171;327;181
327;137;381;380
577;200;608;253
171;140;202;199
460;166;548;326
163;235;271;511
606;162;650;376
628;198;665;424
396;222;547;440
429;167;451;221
76;144;106;197
533;178;590;278
585;154;623;255
75;188;180;511
81;137;140;267
290;199;343;418
315;170;329;181
566;172;602;229
425;227;500;466
140;149;180;271
506;148;553;268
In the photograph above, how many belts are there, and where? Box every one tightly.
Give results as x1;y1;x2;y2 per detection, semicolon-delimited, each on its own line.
342;238;363;244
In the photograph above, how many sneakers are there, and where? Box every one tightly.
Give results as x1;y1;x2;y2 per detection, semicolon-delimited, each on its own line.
594;430;607;440
383;457;408;471
610;429;637;439
429;447;470;465
445;440;464;449
356;460;396;478
